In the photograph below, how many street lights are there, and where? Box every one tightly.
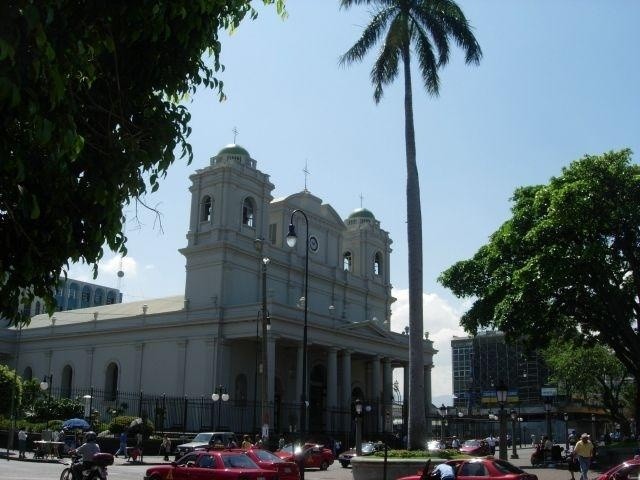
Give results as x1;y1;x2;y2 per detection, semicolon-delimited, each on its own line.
40;374;54;431
286;205;311;447
211;383;230;429
353;395;602;457
254;308;272;441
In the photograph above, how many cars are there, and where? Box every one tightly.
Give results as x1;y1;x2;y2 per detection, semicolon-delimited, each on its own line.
424;441;446;451
221;447;302;480
396;457;538;480
143;450;279;480
338;442;391;468
271;441;334;471
591;454;640;480
457;439;493;455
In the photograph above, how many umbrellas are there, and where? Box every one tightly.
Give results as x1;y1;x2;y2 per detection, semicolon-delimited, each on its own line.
63;418;90;446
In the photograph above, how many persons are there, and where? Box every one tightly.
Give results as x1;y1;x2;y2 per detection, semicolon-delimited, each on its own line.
161;434;171;460
544;436;553;450
115;428;129;458
574;433;594;480
130;447;144;464
55;427;68;458
214;434;286;451
567;431;579;480
452;436;460;449
437;441;446;449
335;440;342;459
432;464;455;480
506;432;512;448
538;437;548;450
486;434;500;456
69;431;100;480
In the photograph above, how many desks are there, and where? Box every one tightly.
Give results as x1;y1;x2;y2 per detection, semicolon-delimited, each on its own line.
33;441;65;460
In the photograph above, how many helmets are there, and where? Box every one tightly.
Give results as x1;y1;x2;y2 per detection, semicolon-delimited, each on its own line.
85;431;97;442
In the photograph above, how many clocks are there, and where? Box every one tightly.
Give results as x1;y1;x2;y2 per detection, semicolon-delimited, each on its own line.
308;235;319;254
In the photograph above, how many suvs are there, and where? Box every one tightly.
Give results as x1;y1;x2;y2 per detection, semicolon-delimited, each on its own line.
174;431;241;462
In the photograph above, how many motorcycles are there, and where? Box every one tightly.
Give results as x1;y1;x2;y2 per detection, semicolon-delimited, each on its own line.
59;449;114;479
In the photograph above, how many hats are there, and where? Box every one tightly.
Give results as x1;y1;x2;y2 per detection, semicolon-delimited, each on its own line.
580;433;590;440
